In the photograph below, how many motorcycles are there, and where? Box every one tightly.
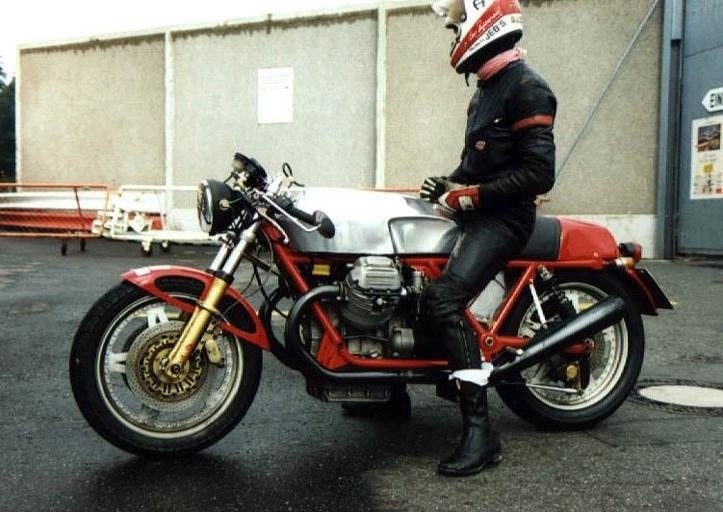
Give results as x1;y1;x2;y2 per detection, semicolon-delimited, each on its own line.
68;153;675;463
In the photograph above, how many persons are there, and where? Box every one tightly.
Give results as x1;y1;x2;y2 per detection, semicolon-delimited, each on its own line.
420;0;556;478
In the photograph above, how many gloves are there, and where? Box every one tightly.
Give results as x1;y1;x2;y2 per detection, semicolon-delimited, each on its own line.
436;180;481;214
419;176;448;205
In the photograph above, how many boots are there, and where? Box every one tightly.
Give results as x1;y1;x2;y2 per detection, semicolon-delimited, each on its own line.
437;361;504;478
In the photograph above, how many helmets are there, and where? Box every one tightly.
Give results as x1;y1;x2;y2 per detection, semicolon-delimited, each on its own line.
429;0;523;74
